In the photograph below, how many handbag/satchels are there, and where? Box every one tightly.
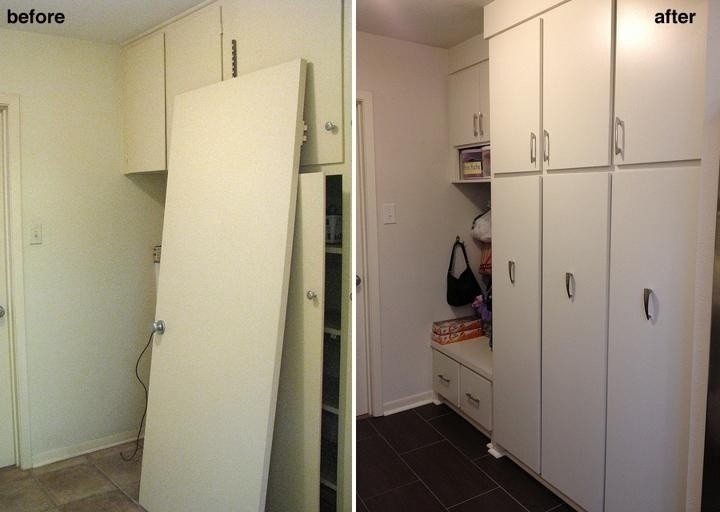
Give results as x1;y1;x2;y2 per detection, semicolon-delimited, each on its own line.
446;241;481;306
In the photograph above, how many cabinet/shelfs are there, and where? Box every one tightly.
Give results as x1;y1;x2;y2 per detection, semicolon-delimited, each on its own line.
445;32;491;184
482;0;720;512
428;335;493;443
264;173;352;512
222;0;353;177
119;1;222;176
